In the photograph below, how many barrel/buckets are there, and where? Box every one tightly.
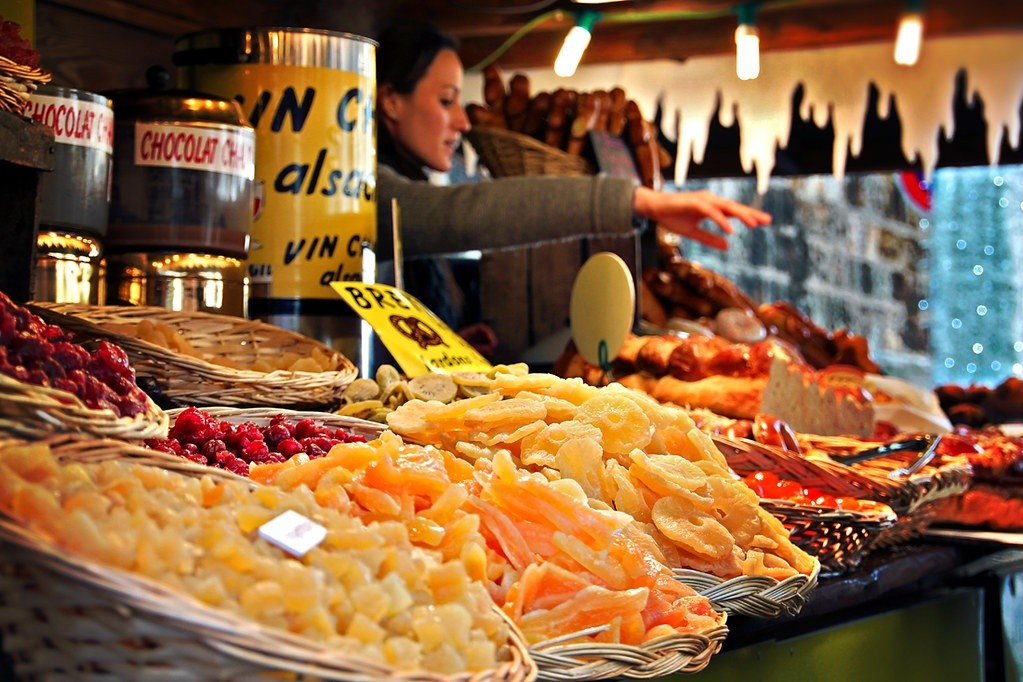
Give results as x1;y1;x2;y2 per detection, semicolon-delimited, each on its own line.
107;84;260;266
169;27;380;317
15;78;114;258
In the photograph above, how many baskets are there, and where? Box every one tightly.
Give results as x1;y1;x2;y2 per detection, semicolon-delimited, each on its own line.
0;298;974;682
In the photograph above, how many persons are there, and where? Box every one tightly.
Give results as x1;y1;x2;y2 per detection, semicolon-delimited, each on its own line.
377;24;774;330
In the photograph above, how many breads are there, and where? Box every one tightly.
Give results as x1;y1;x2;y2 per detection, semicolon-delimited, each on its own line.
565;244;1023;520
